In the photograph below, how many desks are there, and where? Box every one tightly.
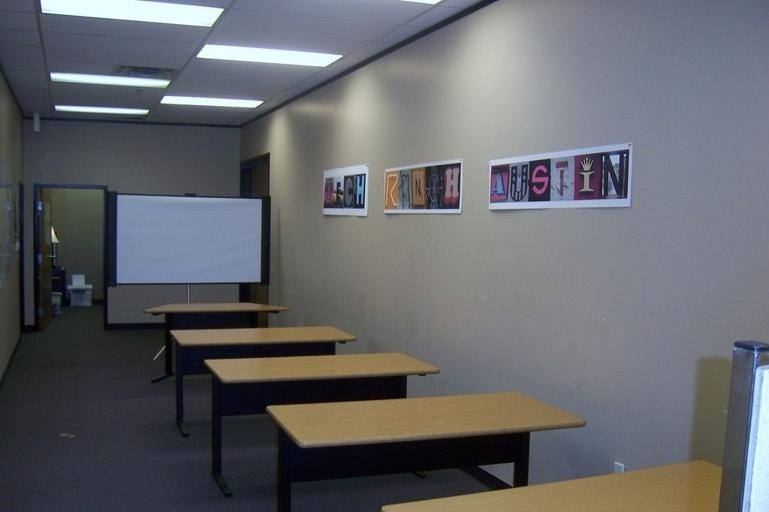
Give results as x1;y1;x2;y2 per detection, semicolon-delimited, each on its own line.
200;351;443;494
267;391;586;510
383;459;725;511
170;323;357;431
143;300;290;386
